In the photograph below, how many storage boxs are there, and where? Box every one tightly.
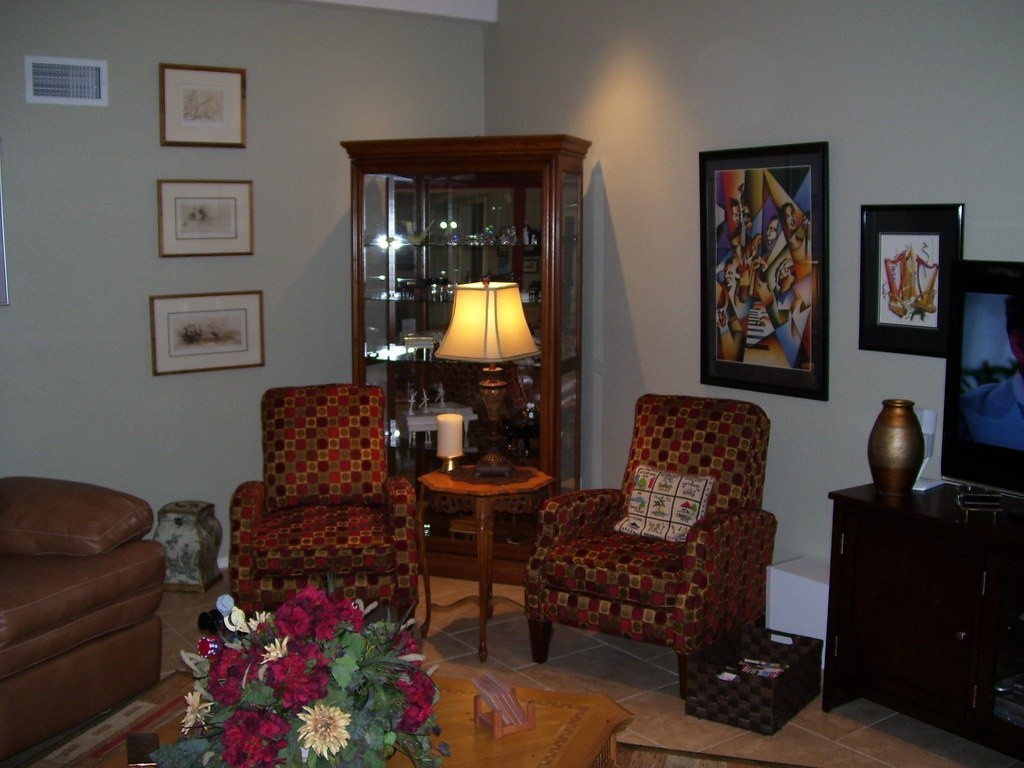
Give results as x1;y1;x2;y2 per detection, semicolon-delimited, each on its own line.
683;628;821;737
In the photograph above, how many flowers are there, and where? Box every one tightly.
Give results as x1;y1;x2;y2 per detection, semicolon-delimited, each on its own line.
145;580;449;768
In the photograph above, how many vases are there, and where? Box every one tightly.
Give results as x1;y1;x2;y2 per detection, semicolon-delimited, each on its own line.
867;398;925;498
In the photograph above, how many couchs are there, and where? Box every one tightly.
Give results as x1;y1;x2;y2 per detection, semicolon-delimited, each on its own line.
0;475;167;762
227;384;418;629
526;394;776;701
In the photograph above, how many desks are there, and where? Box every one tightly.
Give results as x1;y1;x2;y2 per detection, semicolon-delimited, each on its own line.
418;464;554;661
91;673;635;768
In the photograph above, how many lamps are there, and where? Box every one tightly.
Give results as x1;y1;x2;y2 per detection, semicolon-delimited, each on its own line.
434;276;539;479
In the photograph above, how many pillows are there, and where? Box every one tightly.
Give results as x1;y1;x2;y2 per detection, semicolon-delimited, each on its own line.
614;467;715;542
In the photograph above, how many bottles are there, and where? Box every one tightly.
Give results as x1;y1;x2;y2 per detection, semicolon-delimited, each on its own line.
867;399;926;498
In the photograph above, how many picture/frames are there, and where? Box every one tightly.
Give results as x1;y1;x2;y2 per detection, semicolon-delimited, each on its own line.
157;178;255;257
149;290;265;376
158;62;246;148
698;142;831;402
859;203;964;357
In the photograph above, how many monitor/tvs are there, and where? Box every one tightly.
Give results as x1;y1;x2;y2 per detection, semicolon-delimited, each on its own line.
940;264;1024;513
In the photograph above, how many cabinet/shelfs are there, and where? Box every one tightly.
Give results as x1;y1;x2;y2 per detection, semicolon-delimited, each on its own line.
822;480;1024;765
340;133;592;589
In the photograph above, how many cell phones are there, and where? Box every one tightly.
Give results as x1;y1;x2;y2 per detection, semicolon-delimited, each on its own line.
126;733;159;766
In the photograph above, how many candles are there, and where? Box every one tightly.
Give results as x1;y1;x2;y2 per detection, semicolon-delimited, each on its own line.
436;413;464;458
